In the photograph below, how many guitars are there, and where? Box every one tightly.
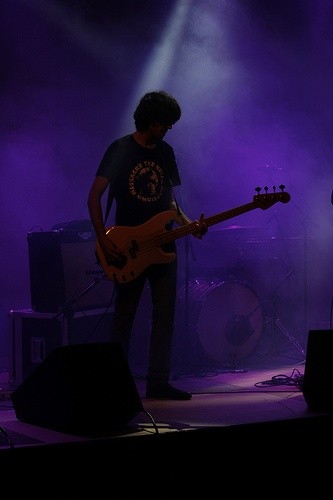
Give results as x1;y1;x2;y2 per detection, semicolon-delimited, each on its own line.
95;183;291;286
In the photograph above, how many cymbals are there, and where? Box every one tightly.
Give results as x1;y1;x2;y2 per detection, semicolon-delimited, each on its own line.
211;225;260;233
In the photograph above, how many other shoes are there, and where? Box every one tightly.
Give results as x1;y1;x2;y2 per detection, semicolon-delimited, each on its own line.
145;383;193;401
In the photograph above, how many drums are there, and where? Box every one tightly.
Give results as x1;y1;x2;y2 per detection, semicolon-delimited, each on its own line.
174;276;266;364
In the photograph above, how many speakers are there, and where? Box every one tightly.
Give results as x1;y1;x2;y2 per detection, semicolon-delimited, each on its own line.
12;338;145;435
304;329;333;412
28;229;123;316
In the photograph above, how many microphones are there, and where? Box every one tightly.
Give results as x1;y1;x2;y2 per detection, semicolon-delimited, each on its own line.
84;267;107;276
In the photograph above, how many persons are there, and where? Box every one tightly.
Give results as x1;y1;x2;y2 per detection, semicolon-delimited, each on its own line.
86;91;208;402
